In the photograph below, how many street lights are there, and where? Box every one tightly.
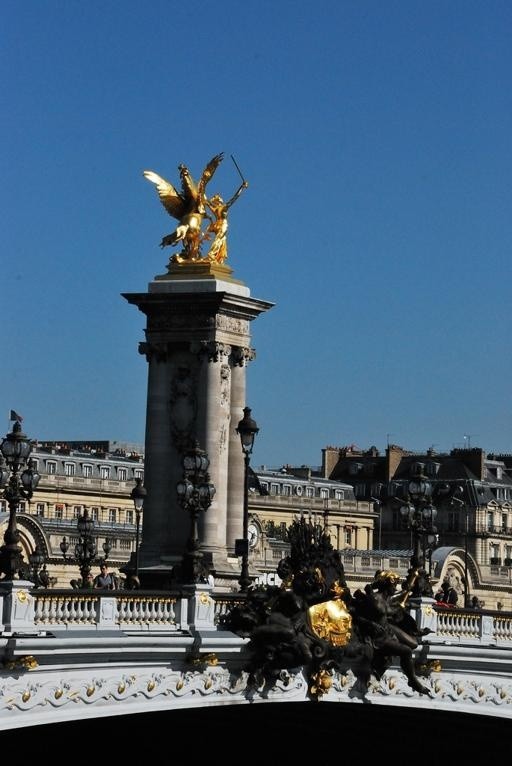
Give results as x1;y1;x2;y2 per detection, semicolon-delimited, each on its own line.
130;477;148;588
369;495;382;550
0;419;41;582
234;407;261;593
176;440;216;585
20;546;49;590
60;510;112;590
449;495;468;609
397;462;439;600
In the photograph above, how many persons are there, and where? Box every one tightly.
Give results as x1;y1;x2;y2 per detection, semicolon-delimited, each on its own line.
205;181;248;264
353;570;431;694
92;563;116;591
441;582;458;605
